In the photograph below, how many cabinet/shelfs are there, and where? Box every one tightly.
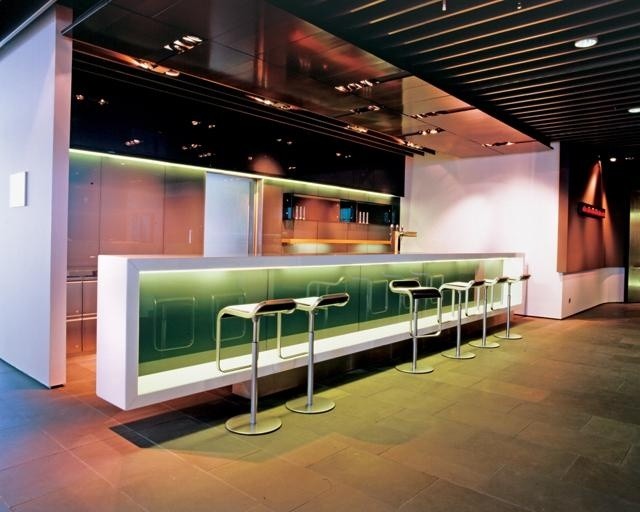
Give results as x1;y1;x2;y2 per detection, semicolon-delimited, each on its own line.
282;192;402;226
67;150;205;357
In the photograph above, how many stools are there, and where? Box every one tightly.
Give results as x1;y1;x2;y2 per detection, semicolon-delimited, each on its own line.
387;274;533;375
215;292;350;436
